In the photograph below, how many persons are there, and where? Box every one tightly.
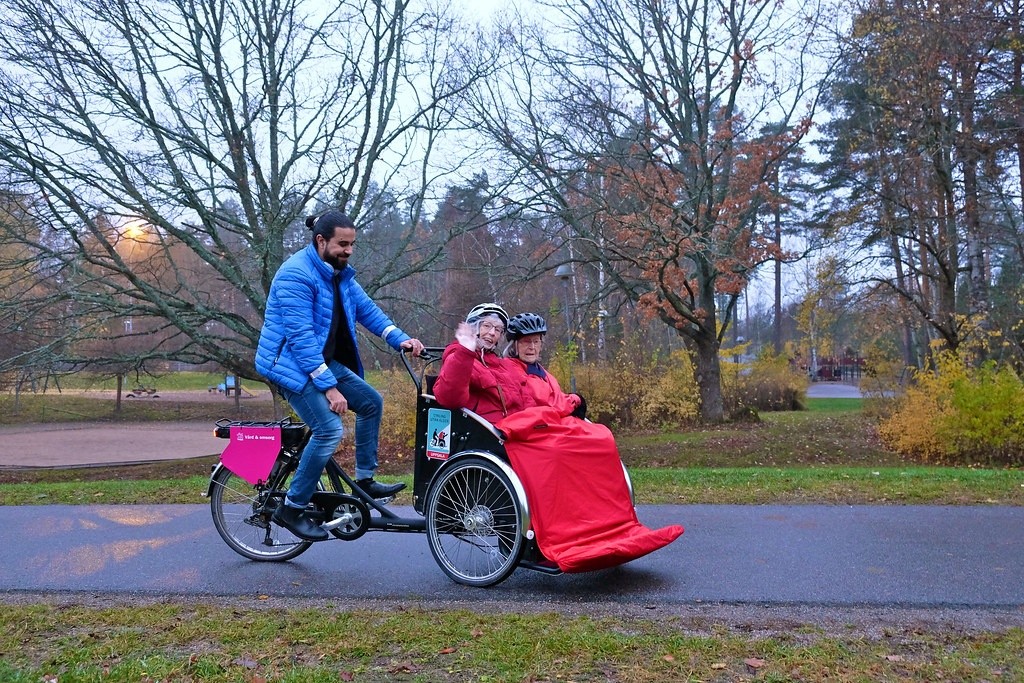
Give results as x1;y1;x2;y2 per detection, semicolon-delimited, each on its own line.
254;210;425;542
433;302;649;566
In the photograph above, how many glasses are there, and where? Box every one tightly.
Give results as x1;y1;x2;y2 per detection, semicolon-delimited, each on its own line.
515;337;544;346
478;320;506;334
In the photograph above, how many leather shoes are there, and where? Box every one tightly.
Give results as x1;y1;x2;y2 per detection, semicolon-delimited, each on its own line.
271;502;332;543
350;476;408;503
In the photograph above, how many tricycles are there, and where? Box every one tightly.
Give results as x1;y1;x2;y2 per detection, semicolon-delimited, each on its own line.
206;346;635;589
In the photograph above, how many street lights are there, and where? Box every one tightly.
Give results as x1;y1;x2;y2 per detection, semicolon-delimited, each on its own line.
554;265;577;394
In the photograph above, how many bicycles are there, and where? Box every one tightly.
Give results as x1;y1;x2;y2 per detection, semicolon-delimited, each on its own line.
125;385;160;398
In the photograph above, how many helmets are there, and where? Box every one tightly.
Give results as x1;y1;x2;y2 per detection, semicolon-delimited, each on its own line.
505;312;547;342
466;302;509;336
